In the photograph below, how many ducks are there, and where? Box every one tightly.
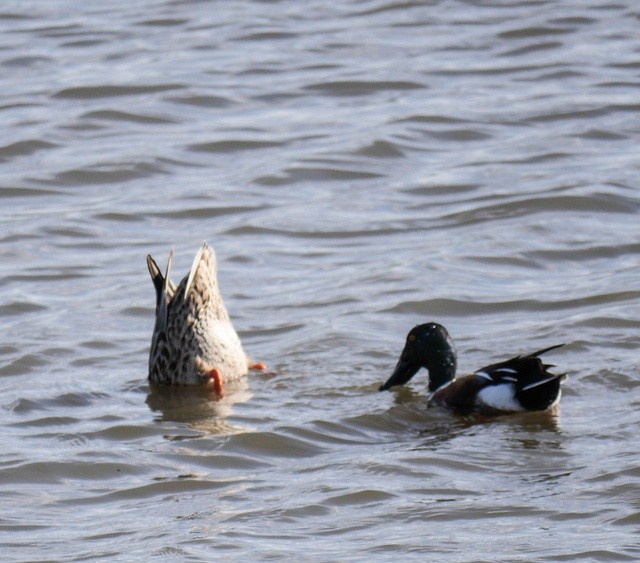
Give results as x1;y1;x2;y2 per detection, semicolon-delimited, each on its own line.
141;244;268;399
380;320;571;430
